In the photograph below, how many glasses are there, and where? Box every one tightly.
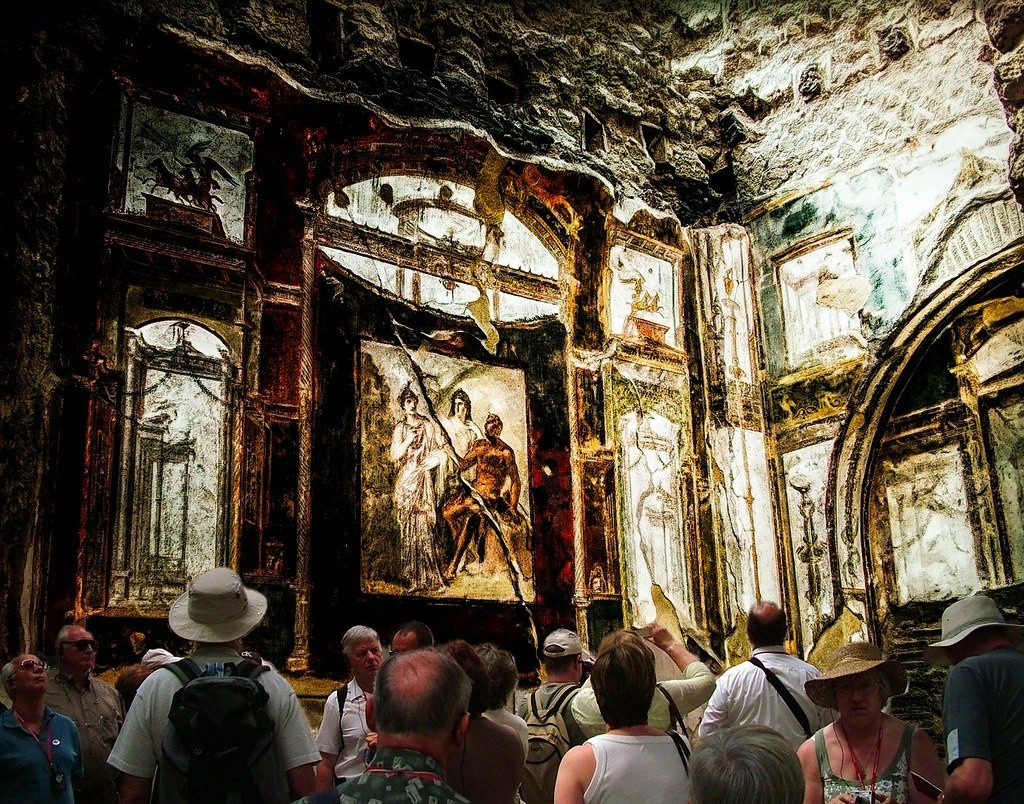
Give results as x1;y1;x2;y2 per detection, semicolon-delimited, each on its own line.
834;679;886;697
7;658;48;679
58;638;99;652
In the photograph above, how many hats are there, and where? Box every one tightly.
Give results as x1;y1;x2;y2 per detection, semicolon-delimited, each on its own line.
803;643;909;707
141;647;185;671
928;595;1024;664
168;567;267;642
542;629;583;658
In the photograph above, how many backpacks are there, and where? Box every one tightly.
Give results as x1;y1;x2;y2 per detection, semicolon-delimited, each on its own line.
515;683;581;804
160;659;283;804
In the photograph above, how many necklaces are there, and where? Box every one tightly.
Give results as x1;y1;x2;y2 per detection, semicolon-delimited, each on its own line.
858;742;877;780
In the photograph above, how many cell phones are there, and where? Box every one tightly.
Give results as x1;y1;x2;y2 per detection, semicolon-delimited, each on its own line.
635;627;652;640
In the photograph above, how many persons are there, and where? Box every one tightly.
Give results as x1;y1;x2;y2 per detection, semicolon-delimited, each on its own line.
517;624;716;804
796;643;946;804
287;622;529;804
688;602;841;804
928;595;1024;804
0;654;84;804
44;625;191;804
107;567;323;804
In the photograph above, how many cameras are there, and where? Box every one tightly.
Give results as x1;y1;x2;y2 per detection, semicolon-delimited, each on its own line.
855;791;876;804
